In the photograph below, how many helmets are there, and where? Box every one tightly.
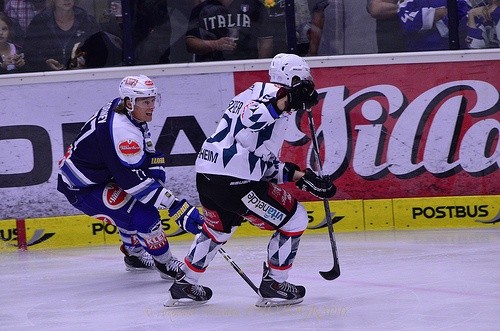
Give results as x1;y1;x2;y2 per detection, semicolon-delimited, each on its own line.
119;75;157;112
269;53;313;86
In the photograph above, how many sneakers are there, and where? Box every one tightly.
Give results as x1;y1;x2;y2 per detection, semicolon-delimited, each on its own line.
152;255;184;280
119;244;157;272
255;261;306;307
163;267;213;308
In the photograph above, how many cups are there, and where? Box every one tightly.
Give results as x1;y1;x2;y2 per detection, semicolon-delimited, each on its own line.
228;26;239;40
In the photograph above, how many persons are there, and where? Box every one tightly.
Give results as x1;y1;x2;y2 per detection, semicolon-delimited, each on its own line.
168;53;338;302
56;74;205;281
0;0;172;75
366;0;500;54
184;0;330;62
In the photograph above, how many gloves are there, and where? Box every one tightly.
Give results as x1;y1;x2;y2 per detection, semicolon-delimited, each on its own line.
147;155;166;183
168;199;203;234
287;79;319;110
294;168;336;199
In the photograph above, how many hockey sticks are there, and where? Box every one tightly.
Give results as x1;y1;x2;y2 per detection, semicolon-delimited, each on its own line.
157;178;261;295
306;108;341;280
